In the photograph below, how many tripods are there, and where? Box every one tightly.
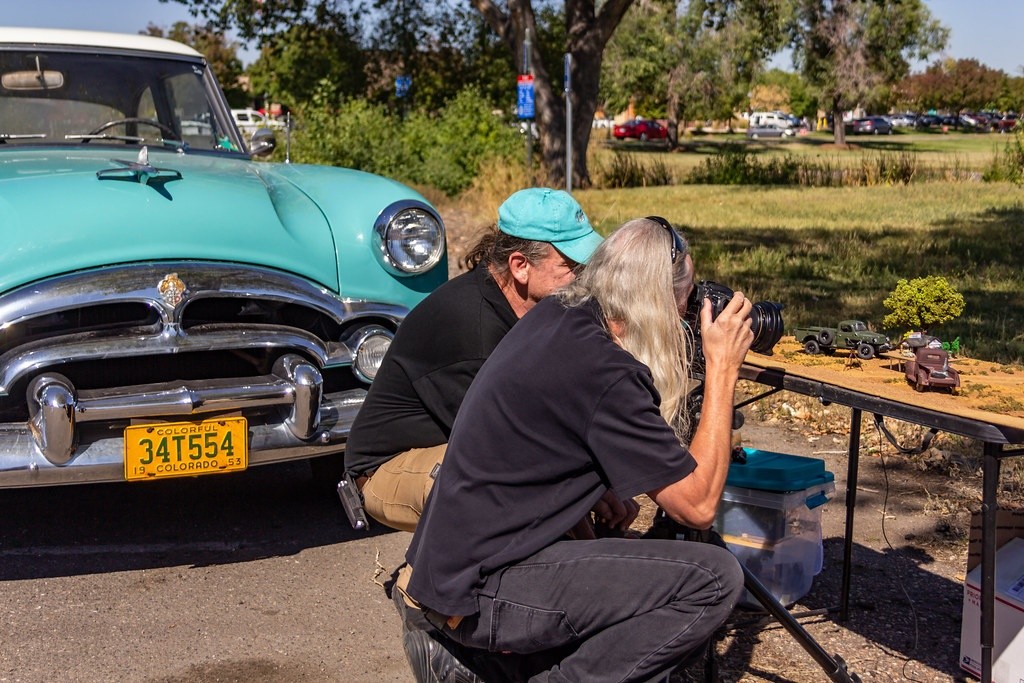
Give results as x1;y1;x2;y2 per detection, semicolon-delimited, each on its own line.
642;379;863;683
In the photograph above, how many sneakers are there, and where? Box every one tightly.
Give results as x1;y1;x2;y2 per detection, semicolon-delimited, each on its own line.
391;582;429;623
403;630;488;683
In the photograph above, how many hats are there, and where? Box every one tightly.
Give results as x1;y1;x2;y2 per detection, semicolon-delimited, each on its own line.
498;187;605;265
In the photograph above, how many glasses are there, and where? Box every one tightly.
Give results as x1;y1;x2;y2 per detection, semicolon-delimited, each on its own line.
645;216;683;263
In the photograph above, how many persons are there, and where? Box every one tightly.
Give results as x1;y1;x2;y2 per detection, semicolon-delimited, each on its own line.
394;215;756;683
347;188;641;633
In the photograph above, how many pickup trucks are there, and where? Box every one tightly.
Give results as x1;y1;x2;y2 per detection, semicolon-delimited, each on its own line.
904;349;962;396
793;320;886;360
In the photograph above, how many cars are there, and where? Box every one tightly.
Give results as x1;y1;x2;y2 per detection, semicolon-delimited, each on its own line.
614;120;666;142
888;110;1018;135
0;26;454;490
748;124;795;140
854;117;892;135
592;117;614;129
231;109;286;136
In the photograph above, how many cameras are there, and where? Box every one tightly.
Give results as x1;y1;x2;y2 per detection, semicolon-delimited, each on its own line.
681;280;785;375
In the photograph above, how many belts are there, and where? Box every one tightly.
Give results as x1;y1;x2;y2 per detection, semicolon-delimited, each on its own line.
426;609;449;630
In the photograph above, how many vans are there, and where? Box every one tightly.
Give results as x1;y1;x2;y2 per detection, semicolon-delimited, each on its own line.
750;112;799;128
